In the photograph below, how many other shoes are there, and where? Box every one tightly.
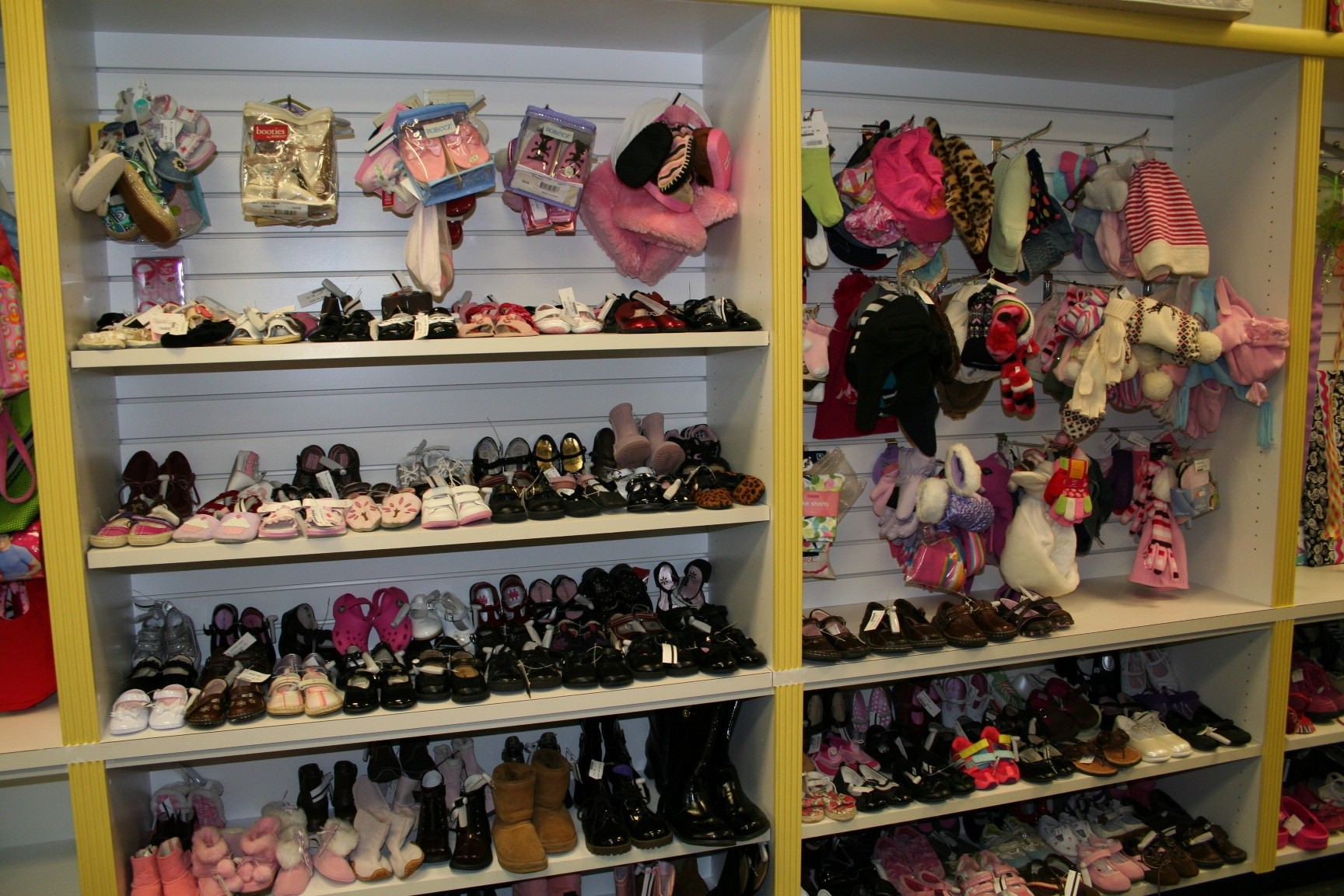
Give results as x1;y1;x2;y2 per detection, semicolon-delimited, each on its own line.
69;92;770;896
801;595;1344;896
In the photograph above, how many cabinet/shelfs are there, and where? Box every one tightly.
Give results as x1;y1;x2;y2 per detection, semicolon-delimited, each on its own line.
0;0;1344;896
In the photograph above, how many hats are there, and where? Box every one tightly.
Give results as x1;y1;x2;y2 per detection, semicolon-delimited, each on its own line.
827;117;1289;597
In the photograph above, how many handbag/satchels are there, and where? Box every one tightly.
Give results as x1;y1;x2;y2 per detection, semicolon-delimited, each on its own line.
0;279;31;395
0;391;40;532
0;579;57;712
